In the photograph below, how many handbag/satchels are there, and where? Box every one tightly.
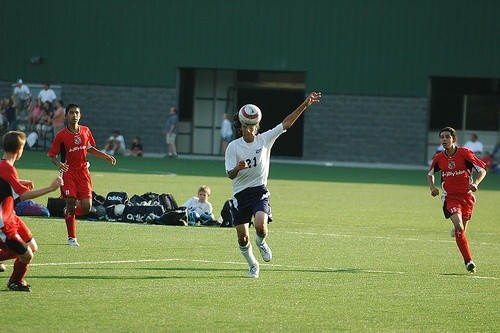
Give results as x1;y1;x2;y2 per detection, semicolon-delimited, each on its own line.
47;197;66;217
91;190;105;206
91;192;165;226
155;207;189;227
103;191;129;205
16;200;50;216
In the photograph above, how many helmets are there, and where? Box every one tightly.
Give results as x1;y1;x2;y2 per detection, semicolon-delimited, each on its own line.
200;211;215;225
188;211;199;223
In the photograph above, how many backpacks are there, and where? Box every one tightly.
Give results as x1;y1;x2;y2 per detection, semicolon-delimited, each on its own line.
160;193;179;210
221;199;240;226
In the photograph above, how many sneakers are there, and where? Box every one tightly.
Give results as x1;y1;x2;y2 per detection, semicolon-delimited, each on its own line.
248;263;260;279
255;240;272;262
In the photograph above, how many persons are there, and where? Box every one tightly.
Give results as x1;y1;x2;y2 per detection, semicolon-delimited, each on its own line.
99;129;126;156
178;186;212;221
428;127;487;273
1;77;67;150
125;136;143;158
0;131;64;294
224;92;323;278
164;108;179;159
46;103;117;245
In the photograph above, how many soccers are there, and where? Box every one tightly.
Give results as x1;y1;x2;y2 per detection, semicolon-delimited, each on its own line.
238;103;262;127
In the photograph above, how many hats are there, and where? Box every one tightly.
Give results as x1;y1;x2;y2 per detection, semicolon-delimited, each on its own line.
112;129;121;135
15;78;23;84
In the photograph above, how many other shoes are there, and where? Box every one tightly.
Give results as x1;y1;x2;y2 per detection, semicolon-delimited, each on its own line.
68;237;79;246
465;261;475;273
0;261;6;272
451;228;455;237
108;150;114;155
6;280;31;292
